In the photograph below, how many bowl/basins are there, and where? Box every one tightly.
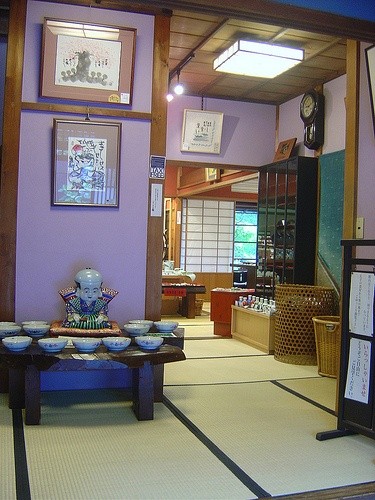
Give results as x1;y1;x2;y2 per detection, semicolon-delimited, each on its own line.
154;321;179;333
135;336;164;350
2;336;32;351
0;321;50;338
124;324;150;336
71;338;101;352
38;338;68;352
102;336;131;351
129;319;154;330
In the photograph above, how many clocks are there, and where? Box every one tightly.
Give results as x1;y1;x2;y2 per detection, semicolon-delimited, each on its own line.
299;88;325;150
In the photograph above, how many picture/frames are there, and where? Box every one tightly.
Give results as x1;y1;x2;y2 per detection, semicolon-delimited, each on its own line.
272;137;297;162
205;168;221;182
51;117;122;209
180;108;225;155
40;15;137;110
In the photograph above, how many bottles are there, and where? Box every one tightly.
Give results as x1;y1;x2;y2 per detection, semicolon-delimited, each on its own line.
238;295;275;313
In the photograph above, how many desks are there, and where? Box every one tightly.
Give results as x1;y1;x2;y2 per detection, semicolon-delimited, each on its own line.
230;304;275;355
0;328;187;425
230;263;256;289
161;285;206;318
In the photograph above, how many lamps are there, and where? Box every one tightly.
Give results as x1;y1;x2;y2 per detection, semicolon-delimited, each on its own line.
212;39;305;79
167;70;185;103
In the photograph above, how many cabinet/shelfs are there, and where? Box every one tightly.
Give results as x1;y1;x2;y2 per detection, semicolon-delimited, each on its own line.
211;290;255;338
255;155;319;298
